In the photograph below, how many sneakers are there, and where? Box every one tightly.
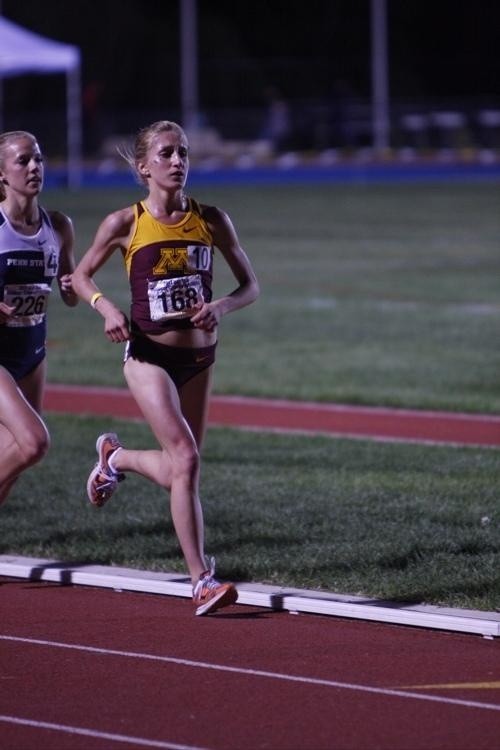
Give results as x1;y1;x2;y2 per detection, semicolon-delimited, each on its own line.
86;432;126;507
192;555;238;617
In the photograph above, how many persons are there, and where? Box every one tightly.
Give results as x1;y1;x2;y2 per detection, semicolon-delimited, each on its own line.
0;131;79;503
71;120;260;616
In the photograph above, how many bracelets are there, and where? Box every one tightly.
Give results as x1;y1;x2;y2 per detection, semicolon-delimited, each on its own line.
90;292;104;309
